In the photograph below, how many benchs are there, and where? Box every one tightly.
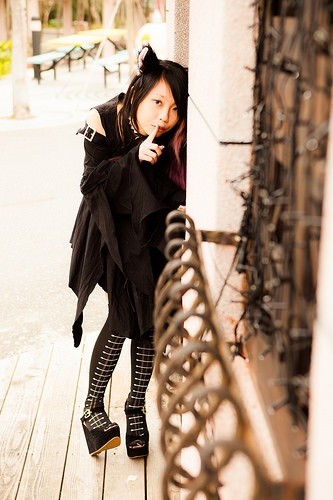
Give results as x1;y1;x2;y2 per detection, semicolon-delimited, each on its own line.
27;46;92;83
97;50;130;87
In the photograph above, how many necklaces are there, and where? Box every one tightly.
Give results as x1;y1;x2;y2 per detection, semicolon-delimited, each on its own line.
128;115;147;138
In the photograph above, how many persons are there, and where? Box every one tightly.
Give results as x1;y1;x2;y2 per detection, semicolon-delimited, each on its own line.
67;46;187;459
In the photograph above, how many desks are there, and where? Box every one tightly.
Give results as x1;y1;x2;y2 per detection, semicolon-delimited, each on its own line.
51;28;129;59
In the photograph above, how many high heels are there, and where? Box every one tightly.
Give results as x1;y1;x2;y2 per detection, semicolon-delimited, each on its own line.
82;405;122;457
124;407;149;459
